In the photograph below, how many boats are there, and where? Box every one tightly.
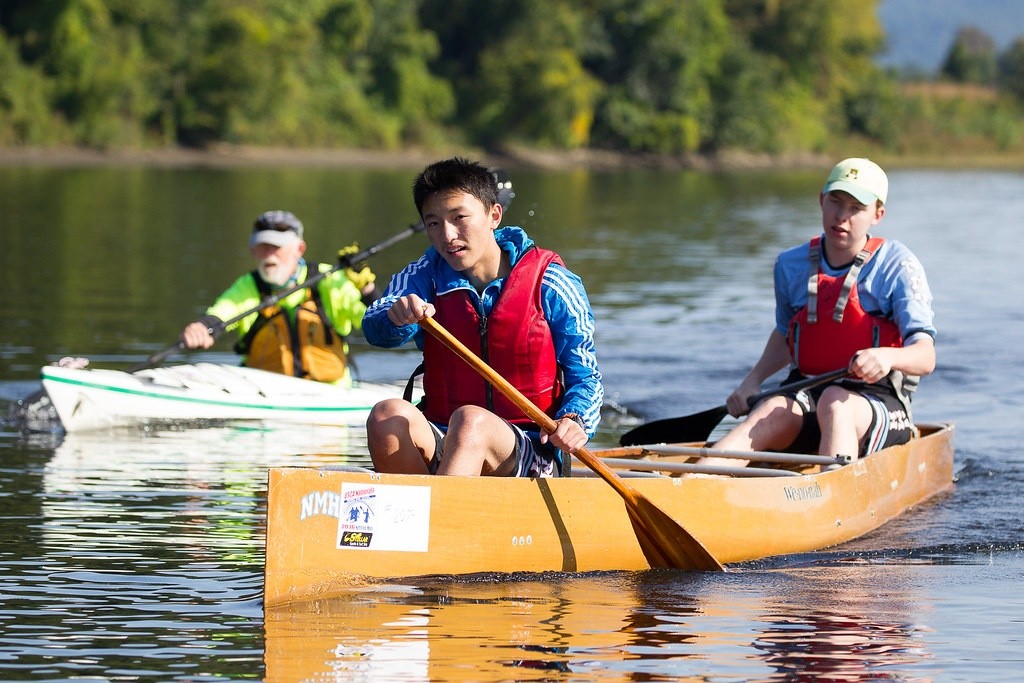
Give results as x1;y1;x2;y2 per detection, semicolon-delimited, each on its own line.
269;419;957;611
44;356;430;434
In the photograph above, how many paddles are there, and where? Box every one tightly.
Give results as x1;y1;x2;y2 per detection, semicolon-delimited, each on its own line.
137;162;520;371
612;349;868;460
403;306;732;586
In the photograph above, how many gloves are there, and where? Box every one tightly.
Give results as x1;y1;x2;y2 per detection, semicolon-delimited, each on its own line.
337;245;376;292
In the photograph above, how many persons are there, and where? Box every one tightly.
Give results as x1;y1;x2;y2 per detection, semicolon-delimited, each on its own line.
362;157;604;476
680;158;937;479
184;210;382;386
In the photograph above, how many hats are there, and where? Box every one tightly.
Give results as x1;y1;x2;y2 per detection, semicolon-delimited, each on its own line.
249;210;303;247
822;158;889;207
